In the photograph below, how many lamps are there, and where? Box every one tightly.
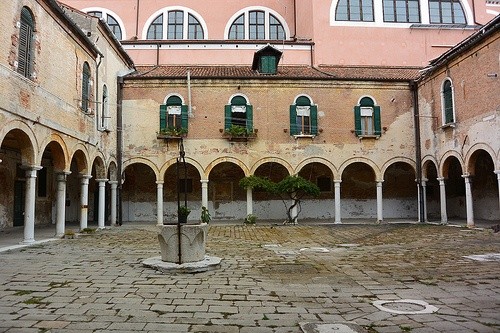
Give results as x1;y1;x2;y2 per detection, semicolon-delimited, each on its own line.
84;31;92;39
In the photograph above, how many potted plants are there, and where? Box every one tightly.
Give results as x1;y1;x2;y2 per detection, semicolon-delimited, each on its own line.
176;205;193;223
